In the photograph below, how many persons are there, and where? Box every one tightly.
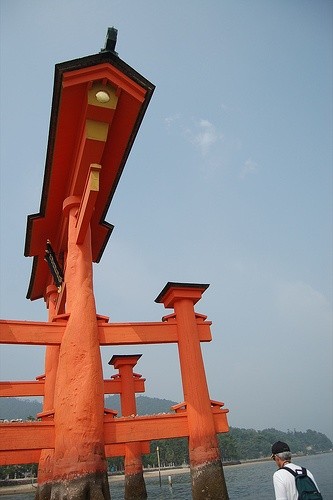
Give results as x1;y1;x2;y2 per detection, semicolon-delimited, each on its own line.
269;440;323;500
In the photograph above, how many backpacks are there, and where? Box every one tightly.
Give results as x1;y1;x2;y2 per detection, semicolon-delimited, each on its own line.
277;466;323;500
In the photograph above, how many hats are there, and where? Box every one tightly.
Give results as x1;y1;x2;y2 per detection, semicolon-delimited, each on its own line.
271;441;291;454
